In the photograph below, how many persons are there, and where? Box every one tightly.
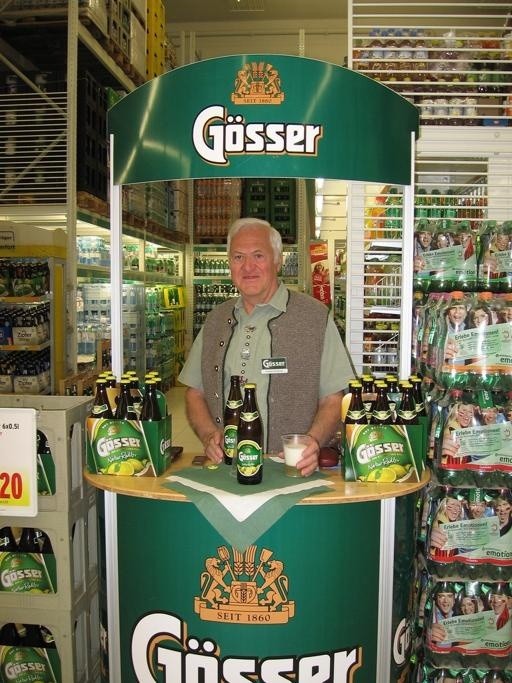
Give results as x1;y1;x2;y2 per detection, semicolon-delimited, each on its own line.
415;587;512;650
414;230;512;281
177;218;356;477
427;402;512;464
415;498;512;563
414;303;512;366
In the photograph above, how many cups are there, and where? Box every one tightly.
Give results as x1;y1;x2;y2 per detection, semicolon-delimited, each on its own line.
282;434;313;477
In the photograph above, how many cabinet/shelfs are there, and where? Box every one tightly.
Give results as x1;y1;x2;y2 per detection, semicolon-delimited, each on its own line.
1;397;106;681
350;0;511;683
198;179;350;405
0;0;196;398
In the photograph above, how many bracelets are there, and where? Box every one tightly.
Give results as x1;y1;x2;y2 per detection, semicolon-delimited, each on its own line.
308;433;322;453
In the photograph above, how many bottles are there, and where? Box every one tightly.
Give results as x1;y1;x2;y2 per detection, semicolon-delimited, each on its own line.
1;256;52;392
193;253;298;340
91;371;168;477
237;384;264;484
67;236;183;393
343;28;511;126
411;185;488;219
332;188;402;370
411;290;512;392
341;373;427;487
413;220;510;291
223;375;243;465
0;426;62;682
411;389;510;489
412;485;511;578
193;179;241;239
412;578;511;681
